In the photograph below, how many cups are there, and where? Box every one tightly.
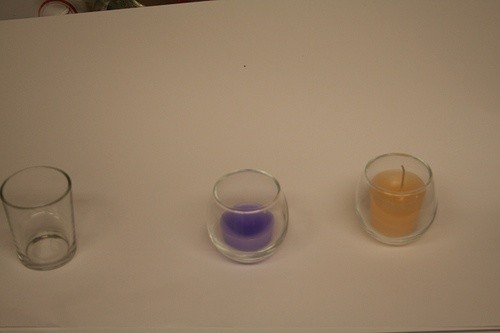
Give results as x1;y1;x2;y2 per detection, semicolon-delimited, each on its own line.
357;153;437;245
207;169;290;264
0;166;78;269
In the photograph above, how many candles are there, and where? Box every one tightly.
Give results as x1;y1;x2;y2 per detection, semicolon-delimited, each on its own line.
357;153;439;245
207;168;290;264
